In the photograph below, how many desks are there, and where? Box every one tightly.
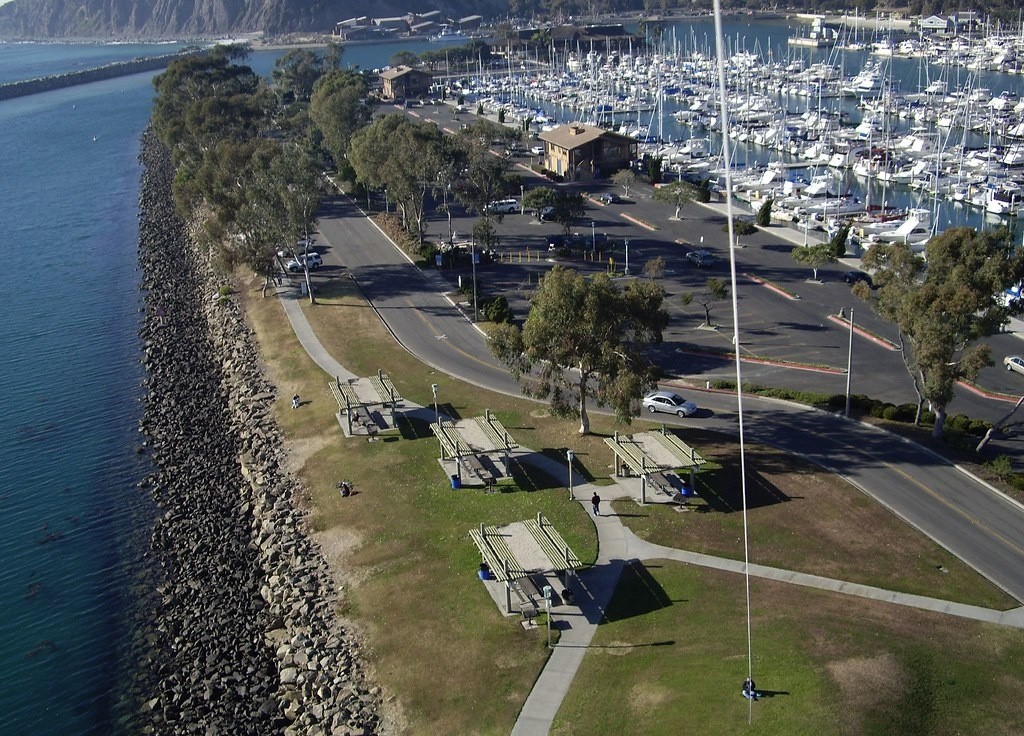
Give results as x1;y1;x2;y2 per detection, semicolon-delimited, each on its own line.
518;602;540;625
482;477;497;491
354;407;370;426
650;472;670;493
515;577;538;602
673;493;688;509
367;427;378;440
464;455;483;474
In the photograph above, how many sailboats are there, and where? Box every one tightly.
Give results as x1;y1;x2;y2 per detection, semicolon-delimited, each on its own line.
430;5;1024;311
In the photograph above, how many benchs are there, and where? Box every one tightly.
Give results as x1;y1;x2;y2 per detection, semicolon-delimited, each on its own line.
364;407;376;424
527;576;544;598
510;582;525;605
477;453;492;470
647;480;659;495
350;409;361;428
660;471;676;491
460;456;472;478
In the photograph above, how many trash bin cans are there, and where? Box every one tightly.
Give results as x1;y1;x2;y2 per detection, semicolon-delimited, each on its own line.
451;474;461;489
681;482;693;497
479;562;490;580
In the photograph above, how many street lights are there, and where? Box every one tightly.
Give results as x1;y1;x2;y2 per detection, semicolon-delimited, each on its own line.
567;450;575;501
592;221;597;256
543;585;552;646
625;238;631;275
432;383;439;422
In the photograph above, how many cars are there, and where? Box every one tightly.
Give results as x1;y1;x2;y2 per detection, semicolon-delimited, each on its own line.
541;207;571;221
841;271;874;290
531;146;544;155
1004;355;1024;375
450;104;470;132
504;147;520;156
600;192;622;203
404;97;439;108
642;390;698;417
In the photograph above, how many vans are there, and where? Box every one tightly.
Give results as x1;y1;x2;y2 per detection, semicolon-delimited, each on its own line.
483;199;519;214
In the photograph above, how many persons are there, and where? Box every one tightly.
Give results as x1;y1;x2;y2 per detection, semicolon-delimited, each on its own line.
591;492;601;516
544;237;550;251
1018;360;1022;365
928;400;933;412
742;677;756;701
277;273;283;287
291;393;300;409
340;482;349;498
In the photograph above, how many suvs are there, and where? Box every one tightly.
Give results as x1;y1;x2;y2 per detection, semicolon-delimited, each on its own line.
285;252;323;273
686;250;715;268
